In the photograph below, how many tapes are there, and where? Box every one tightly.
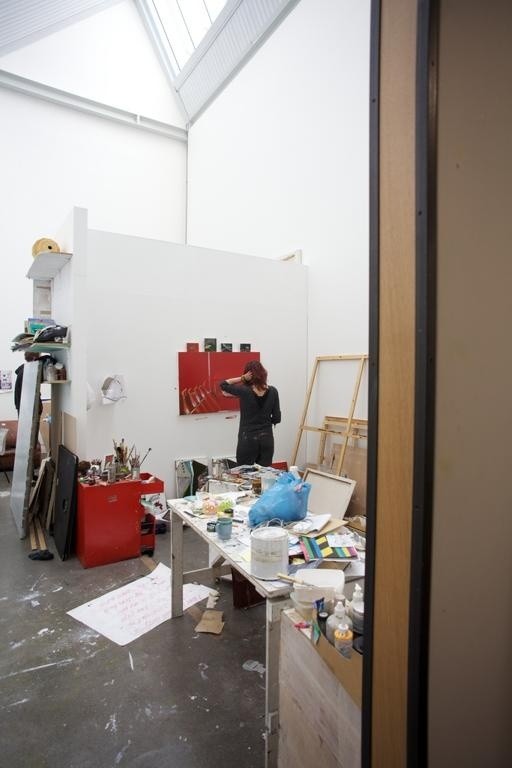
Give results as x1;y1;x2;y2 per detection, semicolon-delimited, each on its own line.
92;476;100;479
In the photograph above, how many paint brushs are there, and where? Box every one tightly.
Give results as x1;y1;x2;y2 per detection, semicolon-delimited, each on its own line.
113;438;151;466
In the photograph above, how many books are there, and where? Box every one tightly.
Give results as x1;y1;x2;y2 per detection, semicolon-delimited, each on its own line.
299;533;361;562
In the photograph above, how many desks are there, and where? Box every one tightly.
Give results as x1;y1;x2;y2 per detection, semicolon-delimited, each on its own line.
167;496;365;768
278;608;362;768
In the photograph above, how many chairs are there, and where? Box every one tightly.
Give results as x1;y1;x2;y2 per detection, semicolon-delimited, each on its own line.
0;429;11;483
0;421;41;470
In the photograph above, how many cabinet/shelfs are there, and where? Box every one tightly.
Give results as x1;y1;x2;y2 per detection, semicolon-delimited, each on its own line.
74;473;164;568
24;342;70;383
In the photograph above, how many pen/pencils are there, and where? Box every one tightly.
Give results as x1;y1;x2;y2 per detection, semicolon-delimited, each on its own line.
92;457;103;465
232;518;243;526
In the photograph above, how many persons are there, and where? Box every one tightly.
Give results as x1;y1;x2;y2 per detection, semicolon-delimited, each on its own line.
219;358;281;467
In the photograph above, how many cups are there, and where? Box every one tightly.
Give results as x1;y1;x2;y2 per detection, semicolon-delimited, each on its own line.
130;467;141;480
215;517;233;540
90;464;101;476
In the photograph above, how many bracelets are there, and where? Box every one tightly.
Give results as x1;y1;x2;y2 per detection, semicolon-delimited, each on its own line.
241;375;249;383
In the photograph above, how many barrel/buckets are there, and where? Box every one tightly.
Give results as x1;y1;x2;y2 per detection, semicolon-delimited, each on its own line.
249;527;290;580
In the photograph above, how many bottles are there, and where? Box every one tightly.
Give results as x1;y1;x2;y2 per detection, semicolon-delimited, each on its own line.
102;472;108;481
251;475;261;492
318;594;365;660
47;363;55;384
224;509;234;518
119;465;128;473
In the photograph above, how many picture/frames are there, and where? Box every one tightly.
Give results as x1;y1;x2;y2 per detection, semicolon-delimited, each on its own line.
302;468;357;519
